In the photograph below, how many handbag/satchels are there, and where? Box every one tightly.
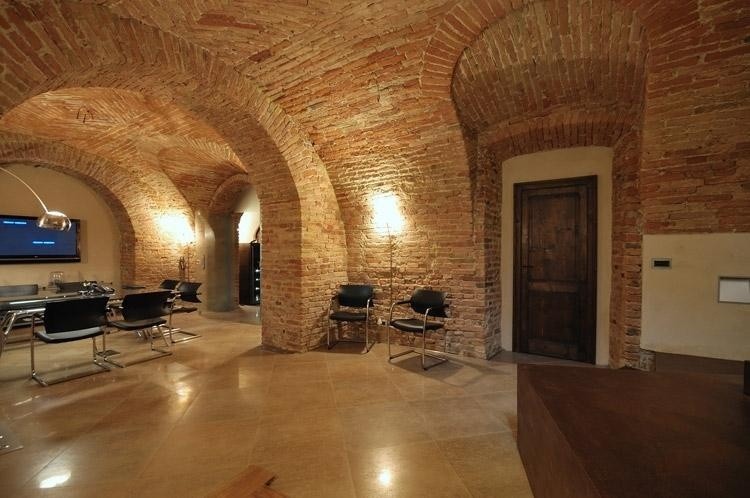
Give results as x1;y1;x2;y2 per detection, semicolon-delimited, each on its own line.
79;282;113;295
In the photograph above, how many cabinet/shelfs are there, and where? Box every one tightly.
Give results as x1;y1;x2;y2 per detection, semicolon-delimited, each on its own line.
250;240;261;304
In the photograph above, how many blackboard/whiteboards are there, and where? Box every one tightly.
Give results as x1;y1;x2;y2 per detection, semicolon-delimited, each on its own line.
641;231;750;361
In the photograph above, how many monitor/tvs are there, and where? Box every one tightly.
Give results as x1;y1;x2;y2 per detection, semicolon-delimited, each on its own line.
0;215;81;264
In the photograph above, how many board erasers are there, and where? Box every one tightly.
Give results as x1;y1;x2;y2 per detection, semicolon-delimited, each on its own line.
655;261;670;266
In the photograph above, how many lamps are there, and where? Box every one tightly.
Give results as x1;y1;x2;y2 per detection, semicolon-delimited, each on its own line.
0;168;72;233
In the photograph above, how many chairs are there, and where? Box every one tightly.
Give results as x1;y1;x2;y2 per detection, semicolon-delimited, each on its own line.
0;281;203;387
326;282;377;354
386;286;451;370
